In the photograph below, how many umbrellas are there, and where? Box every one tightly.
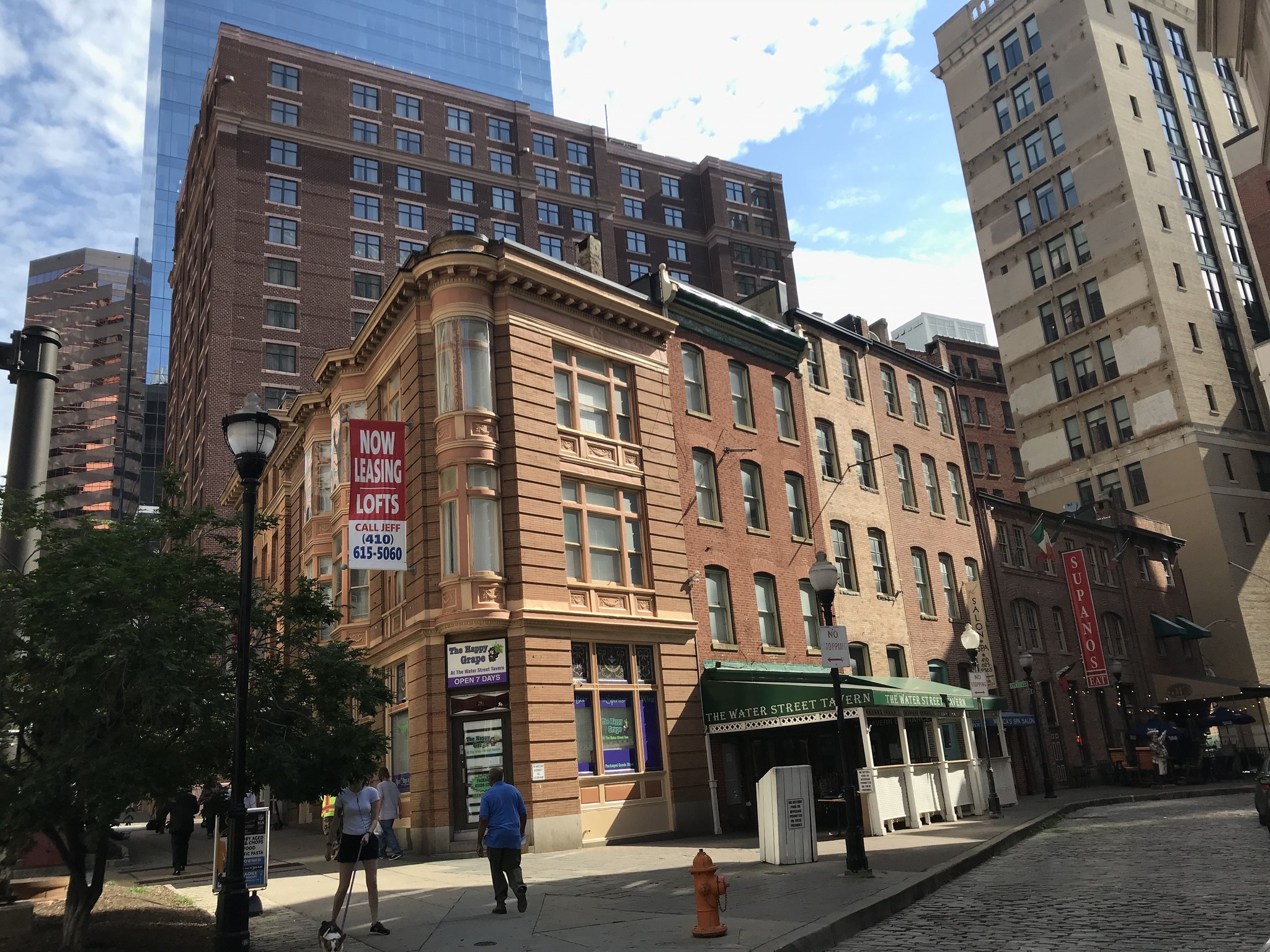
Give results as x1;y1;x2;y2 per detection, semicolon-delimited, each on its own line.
1192;705;1258;751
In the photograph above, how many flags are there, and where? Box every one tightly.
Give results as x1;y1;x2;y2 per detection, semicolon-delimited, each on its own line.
1106;540;1128;569
1030;519;1057;563
1038;526;1061;561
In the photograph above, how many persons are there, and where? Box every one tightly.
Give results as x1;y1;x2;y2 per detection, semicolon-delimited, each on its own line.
224;787;257;809
476;766;527;914
1147;729;1169;786
197;774;223;839
163;780;199;876
151;797;170;834
324;759;391;935
375;766;404;861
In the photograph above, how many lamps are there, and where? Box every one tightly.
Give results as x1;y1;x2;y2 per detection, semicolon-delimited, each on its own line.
837;577;841;586
689;570;700;583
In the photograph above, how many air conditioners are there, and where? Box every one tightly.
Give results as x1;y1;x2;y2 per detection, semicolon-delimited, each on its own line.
1137;548;1149;557
1161;551;1168;561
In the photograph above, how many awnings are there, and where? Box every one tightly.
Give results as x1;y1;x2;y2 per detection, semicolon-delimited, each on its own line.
1176;617;1213;641
1153;671;1269;706
970;711;1036;728
1151;612;1187;640
695;658;1009;723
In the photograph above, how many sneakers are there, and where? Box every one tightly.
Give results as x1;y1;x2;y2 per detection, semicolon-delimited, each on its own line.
370;920;390;935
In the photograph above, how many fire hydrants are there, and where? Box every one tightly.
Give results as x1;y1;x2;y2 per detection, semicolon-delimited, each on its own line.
688;849;730;937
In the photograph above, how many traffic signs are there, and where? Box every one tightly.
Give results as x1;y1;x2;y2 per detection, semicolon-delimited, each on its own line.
818;624;850;667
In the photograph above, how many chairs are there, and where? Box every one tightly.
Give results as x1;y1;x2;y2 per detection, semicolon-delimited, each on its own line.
1075;746;1175;786
814;772;843;821
1188;753;1237;784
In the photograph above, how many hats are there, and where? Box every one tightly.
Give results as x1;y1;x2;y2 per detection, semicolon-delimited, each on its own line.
380;766;390;777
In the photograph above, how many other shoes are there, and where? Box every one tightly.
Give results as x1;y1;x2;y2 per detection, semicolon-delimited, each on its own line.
207;833;213;838
516;887;527;912
180;866;185;871
156;827;165;834
492;905;508;914
378;856;385;859
388;854;403;860
172;870;180;876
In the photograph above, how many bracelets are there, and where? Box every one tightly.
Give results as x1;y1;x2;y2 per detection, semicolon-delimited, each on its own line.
477;844;483;848
326;843;332;847
366;831;372;835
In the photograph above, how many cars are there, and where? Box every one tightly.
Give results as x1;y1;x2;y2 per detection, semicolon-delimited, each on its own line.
1254;758;1270;830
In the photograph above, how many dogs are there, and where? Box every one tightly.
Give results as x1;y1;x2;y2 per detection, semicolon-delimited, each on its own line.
317;921;348;952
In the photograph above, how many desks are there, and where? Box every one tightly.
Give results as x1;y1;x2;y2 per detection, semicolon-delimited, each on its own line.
818;799;846;837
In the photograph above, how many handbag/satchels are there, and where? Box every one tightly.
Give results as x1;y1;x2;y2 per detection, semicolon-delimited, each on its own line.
146;819;158;830
201;820;207;828
373;820;384;838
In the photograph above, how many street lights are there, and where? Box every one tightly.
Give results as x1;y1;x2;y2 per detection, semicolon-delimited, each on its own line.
807;551;877;879
209;390;281;952
1110;659;1140;764
1017;648;1058;798
959;622;1005;818
